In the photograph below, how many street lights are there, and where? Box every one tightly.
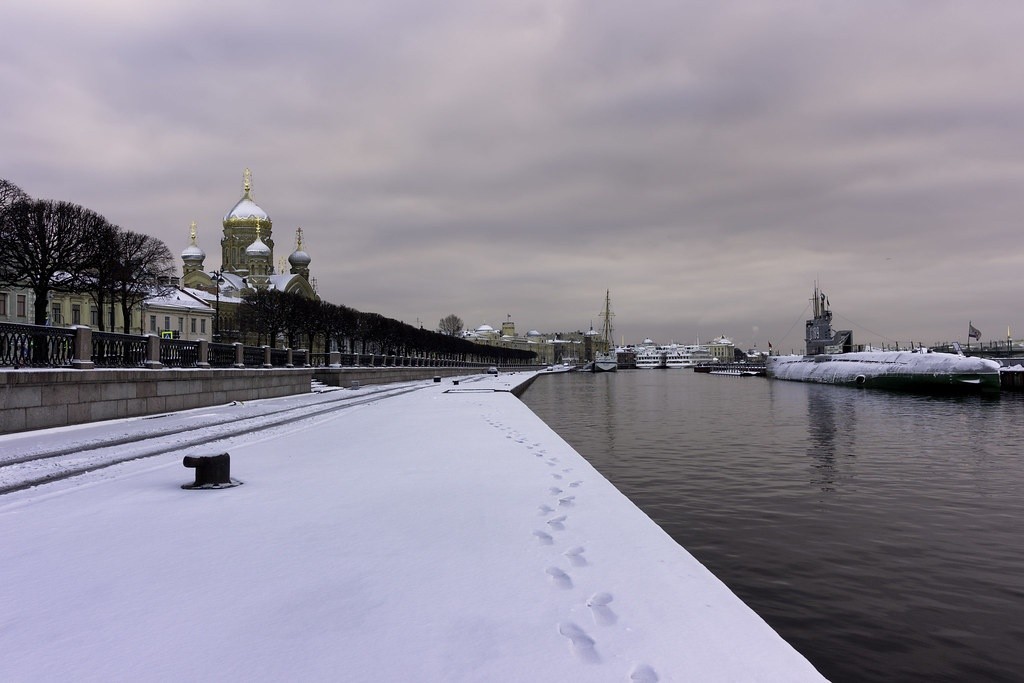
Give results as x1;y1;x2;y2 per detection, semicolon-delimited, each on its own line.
210;264;225;341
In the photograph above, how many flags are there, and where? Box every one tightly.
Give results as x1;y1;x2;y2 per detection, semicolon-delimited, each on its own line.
969;322;982;341
508;315;511;317
769;342;772;348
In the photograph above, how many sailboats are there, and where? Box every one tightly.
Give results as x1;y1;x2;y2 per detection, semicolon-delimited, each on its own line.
594;288;618;372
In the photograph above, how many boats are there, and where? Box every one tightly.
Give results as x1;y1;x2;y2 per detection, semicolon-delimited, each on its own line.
667;350;713;369
635;351;666;369
